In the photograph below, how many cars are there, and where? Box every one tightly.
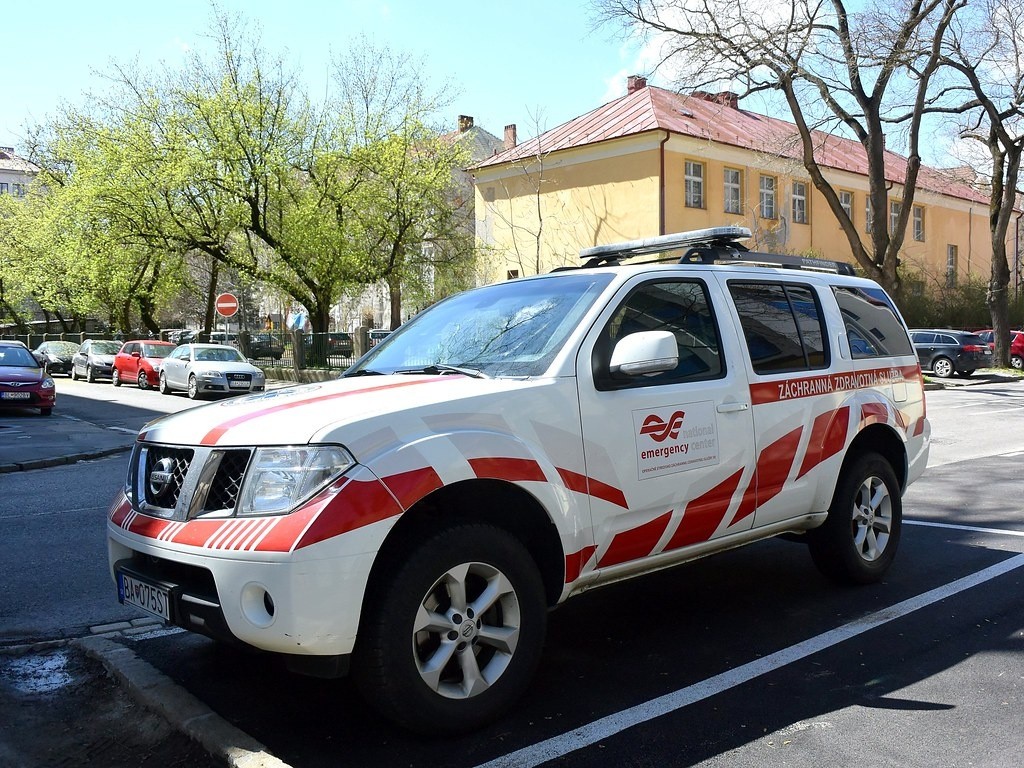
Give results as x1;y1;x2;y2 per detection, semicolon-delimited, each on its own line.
71;338;124;383
159;343;266;398
32;341;80;377
111;340;177;388
251;332;286;360
0;340;58;417
153;330;240;346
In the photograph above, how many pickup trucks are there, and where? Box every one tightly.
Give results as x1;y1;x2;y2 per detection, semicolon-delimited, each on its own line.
908;329;993;380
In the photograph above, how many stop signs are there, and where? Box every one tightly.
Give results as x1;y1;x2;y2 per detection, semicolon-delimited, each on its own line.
215;292;239;317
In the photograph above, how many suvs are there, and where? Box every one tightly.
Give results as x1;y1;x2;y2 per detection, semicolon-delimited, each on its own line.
327;330;353;358
973;330;1024;370
105;227;933;742
